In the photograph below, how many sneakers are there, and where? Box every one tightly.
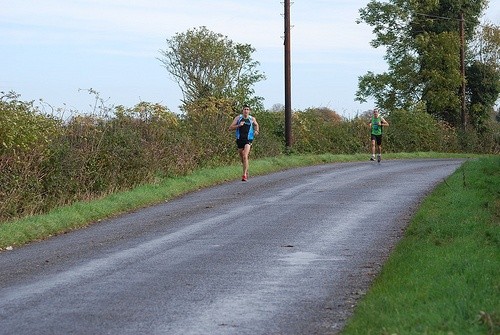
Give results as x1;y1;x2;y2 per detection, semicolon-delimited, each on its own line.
242;175;248;181
370;156;376;161
377;155;382;163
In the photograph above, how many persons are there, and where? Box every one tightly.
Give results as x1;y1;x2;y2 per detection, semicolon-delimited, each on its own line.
229;106;259;181
368;109;389;162
